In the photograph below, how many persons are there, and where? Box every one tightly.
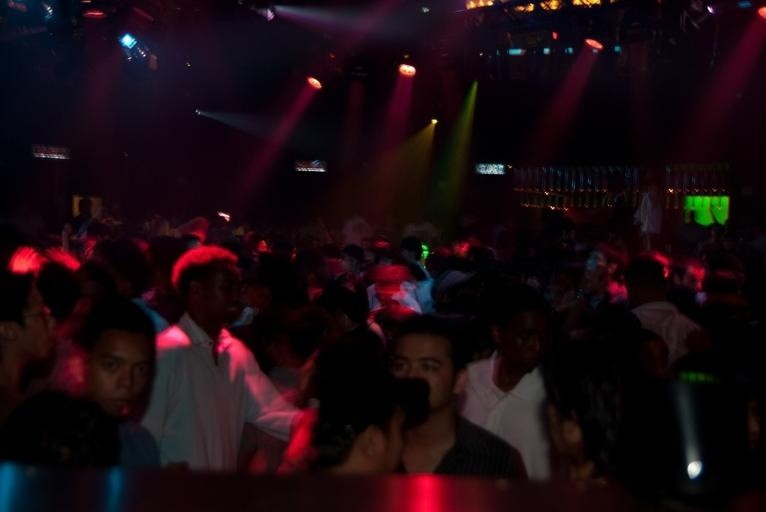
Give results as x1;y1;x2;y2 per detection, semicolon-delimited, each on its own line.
0;166;766;512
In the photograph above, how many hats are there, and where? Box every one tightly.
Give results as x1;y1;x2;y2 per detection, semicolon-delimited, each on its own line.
321;366;431;426
